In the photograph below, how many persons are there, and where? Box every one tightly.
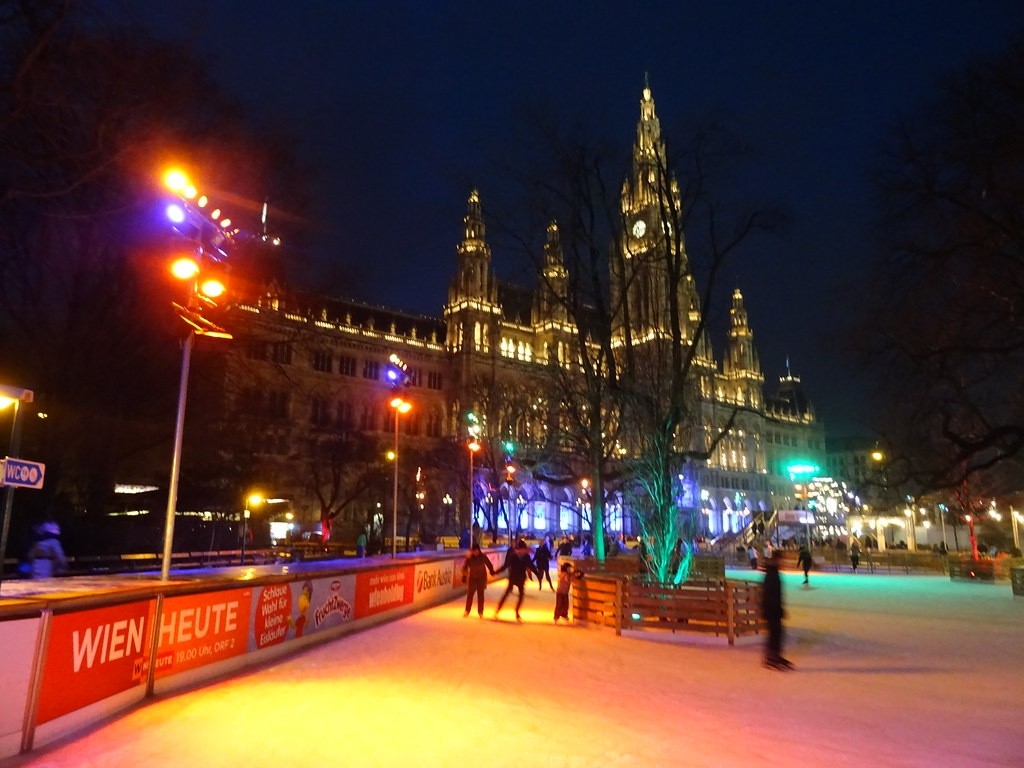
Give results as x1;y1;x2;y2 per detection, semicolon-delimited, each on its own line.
761;552;793;672
752;520;764;536
849;542;860;574
496;540;540;620
554;563;580;621
609;532;626;557
555;537;572;557
461;543;495;620
797;547;812;583
581;537;590;556
533;538;554;592
31;521;65;578
748;545;759;569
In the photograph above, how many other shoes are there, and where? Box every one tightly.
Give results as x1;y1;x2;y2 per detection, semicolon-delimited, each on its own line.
765;654;792;671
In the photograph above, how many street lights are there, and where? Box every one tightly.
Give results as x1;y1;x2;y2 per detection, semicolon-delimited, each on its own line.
143;164;235;582
788;461;818;553
465;409;484;542
240;495;260;565
385;353;415;560
502;440;516;549
0;383;34;578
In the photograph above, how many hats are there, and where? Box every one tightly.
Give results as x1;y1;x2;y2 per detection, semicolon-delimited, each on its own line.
40;521;60;535
